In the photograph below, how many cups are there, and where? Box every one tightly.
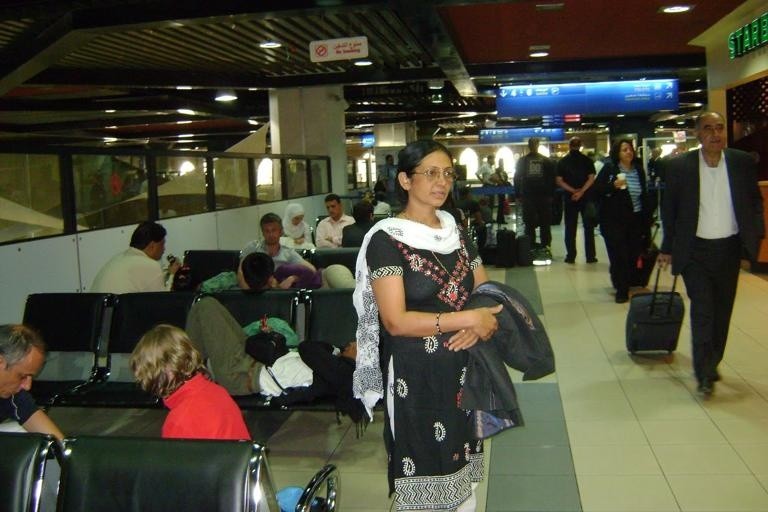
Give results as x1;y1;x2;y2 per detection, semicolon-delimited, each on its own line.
616;173;627;190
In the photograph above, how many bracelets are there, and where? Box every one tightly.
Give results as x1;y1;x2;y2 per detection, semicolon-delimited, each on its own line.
436;312;445;335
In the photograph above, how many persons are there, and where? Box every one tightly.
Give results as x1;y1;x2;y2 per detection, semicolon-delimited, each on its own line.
315;194;357;248
184;296;385;407
659;113;765;397
128;322;251;440
237;203;318;290
91;221;182;294
353;141;504;512
555;136;606;264
371;154;401;215
475;155;509;224
445;186;493;252
342;200;376;248
514;137;556;249
0;325;64;443
588;139;664;304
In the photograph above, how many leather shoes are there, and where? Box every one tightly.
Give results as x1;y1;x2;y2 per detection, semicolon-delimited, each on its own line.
699;377;713;392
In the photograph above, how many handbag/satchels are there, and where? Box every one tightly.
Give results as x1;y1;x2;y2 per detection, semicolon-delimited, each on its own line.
245;331;289;367
584;202;600;226
482;229;552;267
374;182;386;192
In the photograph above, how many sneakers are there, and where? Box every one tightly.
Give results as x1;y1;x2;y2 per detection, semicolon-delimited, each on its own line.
565;258;575;263
586;258;597;263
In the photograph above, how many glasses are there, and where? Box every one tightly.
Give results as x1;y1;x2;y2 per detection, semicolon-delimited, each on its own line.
407;166;460;183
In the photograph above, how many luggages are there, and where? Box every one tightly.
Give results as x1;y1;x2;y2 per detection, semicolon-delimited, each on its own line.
610;223;659;288
626;260;684;355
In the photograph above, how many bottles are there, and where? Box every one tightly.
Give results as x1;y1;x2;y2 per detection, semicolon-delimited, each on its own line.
605;175;614;197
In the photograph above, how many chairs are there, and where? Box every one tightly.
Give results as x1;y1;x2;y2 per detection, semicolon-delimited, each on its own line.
175;214;365;284
80;289;198;410
54;435;344;512
202;287;299;410
1;429;59;512
17;290;111;404
272;285;371;433
